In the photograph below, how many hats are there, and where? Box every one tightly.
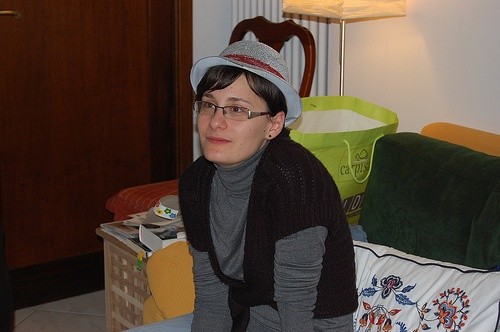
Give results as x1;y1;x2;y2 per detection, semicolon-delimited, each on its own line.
191;40;302;127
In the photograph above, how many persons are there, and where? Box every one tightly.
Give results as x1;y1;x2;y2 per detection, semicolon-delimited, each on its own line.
178;41;359;332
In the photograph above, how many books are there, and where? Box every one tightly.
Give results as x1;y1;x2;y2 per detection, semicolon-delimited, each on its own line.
101;210;187;258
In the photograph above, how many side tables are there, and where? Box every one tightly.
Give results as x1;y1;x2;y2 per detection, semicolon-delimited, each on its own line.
95;220;188;332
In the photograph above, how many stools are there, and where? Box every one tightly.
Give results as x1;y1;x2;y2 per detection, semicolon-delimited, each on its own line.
105;179;178;220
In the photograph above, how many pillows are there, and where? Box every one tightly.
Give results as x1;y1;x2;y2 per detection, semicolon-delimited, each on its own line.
353;241;500;332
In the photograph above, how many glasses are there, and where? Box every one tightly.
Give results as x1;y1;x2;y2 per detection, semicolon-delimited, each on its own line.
193;101;270;121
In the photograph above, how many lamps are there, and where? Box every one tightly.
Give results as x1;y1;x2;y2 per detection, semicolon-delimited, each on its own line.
282;0;406;97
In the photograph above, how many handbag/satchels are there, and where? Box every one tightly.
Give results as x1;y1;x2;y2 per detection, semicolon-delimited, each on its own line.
286;96;400;226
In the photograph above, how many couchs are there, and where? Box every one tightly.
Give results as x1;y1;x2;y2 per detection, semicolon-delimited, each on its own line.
141;121;500;332
